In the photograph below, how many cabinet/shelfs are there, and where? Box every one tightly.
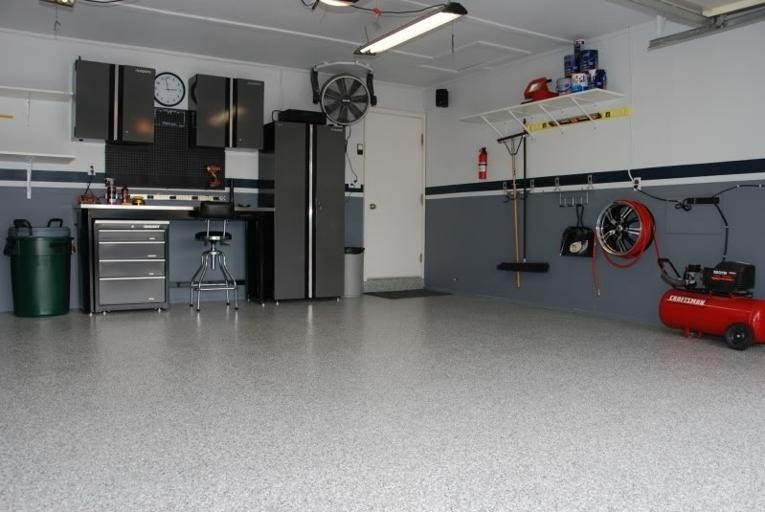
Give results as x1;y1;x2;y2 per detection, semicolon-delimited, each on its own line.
78;204;275;317
188;74;264;150
71;60;155;144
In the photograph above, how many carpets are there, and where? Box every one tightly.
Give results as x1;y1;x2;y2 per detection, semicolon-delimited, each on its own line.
362;289;453;300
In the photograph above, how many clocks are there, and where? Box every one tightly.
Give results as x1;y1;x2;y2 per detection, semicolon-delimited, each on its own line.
154;72;186;107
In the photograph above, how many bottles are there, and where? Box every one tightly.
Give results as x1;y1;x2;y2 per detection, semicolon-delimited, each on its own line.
108;178;117;203
122;184;130;203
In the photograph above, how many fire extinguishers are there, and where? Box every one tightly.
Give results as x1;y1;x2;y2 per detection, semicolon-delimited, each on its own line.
478;147;488;179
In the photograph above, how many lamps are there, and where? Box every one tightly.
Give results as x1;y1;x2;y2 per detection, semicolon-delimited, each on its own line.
353;2;468;56
301;0;360;11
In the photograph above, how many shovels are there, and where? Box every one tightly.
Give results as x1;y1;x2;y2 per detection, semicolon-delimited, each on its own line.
559;205;594;257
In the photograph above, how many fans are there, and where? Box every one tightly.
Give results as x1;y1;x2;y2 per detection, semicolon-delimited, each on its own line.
310;60;377;126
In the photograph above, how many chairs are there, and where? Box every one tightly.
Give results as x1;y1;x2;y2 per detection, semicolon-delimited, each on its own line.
190;201;239;313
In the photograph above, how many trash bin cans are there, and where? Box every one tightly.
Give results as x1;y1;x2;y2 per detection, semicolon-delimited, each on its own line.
341;247;365;297
